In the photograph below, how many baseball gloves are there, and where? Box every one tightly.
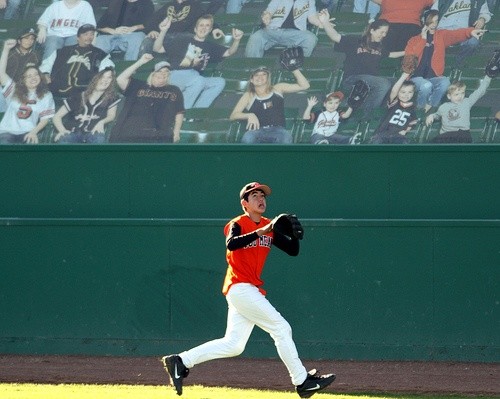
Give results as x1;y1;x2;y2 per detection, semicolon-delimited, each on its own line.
348;80;370;106
484;50;500;78
280;47;306;71
270;212;303;241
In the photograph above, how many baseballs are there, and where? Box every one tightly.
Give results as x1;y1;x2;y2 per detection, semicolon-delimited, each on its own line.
354;95;361;102
290;59;297;64
491;66;498;70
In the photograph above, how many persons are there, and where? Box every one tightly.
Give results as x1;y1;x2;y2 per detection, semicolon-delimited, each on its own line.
52;63;123;144
137;0;198;60
200;0;254;14
321;0;497;68
244;0;339;59
317;11;405;110
404;10;488;109
94;0;155;61
49;22;114;108
227;45;311;144
423;73;492;143
109;54;186;143
162;182;336;399
302;80;368;145
371;54;422;144
5;27;40;85
0;37;56;143
35;0;97;83
152;14;243;109
0;0;27;22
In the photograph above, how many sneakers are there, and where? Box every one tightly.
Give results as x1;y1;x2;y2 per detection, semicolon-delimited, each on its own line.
296;369;336;399
163;354;189;396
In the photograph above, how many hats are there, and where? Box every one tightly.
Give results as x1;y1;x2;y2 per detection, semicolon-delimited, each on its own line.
250;66;270;79
20;27;38;38
154;61;170;71
78;24;96;36
240;182;271;199
326;90;346;101
